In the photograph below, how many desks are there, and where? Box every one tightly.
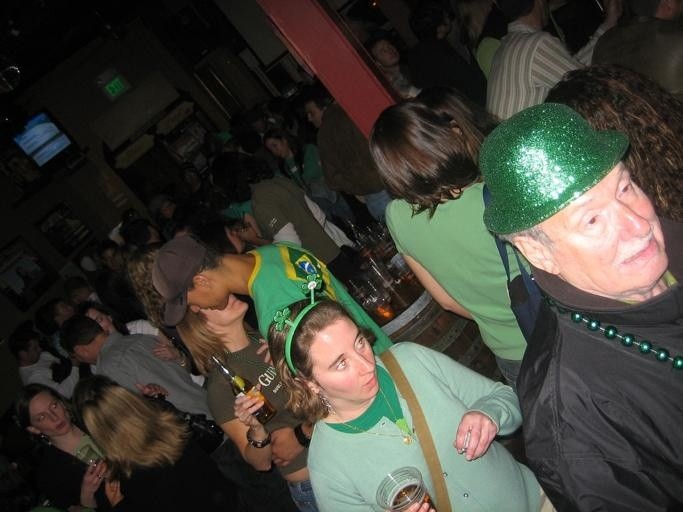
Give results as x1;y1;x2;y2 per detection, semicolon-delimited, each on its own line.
339;239;485;371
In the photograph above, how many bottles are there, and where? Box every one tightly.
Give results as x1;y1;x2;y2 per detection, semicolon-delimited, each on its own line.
208;353;277;425
342;215;369;249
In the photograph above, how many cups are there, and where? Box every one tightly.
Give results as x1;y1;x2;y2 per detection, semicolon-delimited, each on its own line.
344;246;415;323
74;444;112;481
360;215;396;245
375;465;437;511
238;222;249;232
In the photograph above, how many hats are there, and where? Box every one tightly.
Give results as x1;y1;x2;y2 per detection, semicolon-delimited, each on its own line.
149;229;209;327
473;100;633;237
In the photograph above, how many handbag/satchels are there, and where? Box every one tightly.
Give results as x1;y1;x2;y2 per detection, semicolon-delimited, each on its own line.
154;402;228;480
